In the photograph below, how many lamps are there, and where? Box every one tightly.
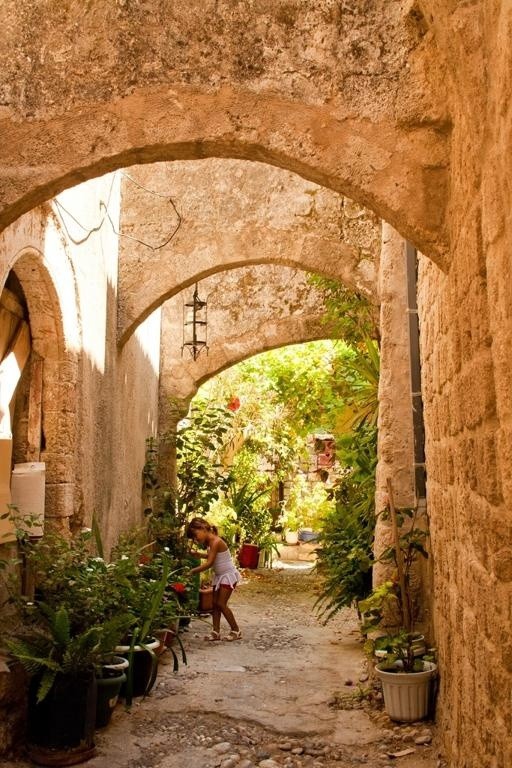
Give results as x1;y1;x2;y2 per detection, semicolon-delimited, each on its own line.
181;280;208;361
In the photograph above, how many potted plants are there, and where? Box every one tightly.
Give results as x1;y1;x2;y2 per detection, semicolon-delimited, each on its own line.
238;500;279;567
358;477;441;723
0;503;213;768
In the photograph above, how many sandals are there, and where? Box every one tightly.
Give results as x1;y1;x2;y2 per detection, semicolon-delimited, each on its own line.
221;627;242;641
204;630;221;641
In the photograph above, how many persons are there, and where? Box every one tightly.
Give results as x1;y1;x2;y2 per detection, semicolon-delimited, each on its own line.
186;516;242;641
